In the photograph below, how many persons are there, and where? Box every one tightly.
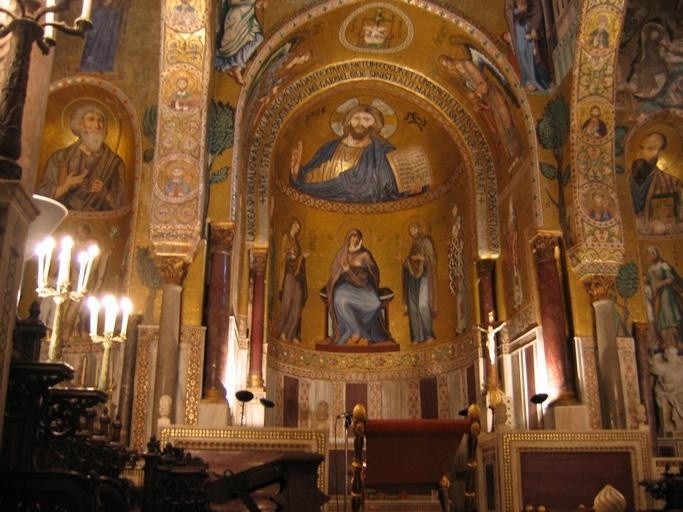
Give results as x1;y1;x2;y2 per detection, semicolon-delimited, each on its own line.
243;50;315;147
438;56;525;165
628;131;683;434
500;1;556;93
165;0;198;199
581;102;608;140
269;198;467;346
76;0;129;74
74;225;99;249
588;16;614;51
590;189;611;221
216;0;268;86
36;104;128;212
289;103;428;203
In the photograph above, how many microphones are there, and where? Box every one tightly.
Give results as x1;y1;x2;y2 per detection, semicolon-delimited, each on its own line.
337;412;353;418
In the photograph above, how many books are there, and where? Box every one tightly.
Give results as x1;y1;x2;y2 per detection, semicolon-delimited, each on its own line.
385;146;434;193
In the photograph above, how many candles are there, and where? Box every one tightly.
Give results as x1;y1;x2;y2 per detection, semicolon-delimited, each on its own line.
79;0;92;20
34;232;104;295
43;0;57;44
89;295;133;339
